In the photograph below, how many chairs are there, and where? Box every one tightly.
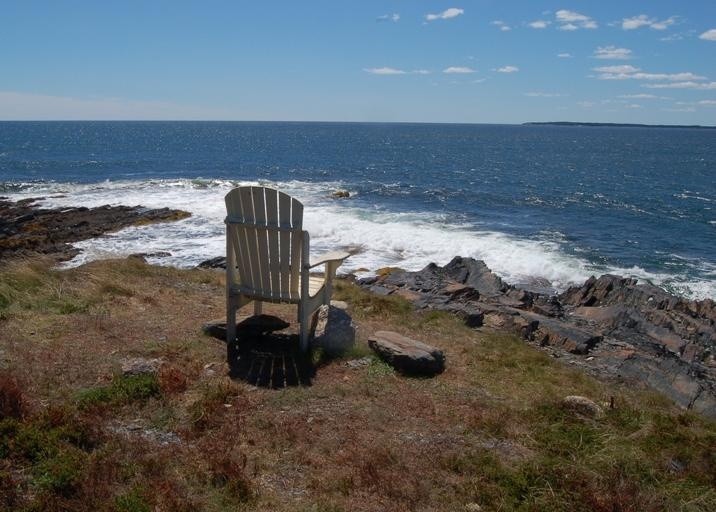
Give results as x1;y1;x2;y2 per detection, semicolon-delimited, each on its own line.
223;187;350;356
